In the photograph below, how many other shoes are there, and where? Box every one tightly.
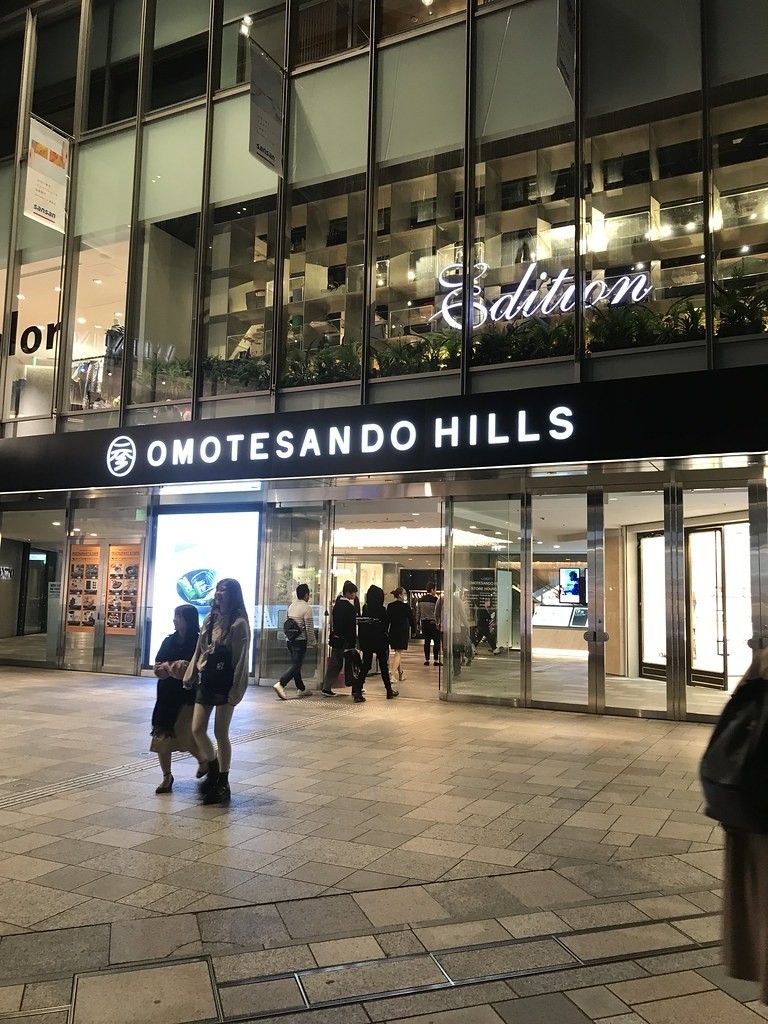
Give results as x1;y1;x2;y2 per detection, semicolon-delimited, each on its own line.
434;662;443;665
455;670;461;676
390;675;397;683
399;671;406;680
354;696;366;703
362;689;366;694
321;689;338;697
424;662;429;665
388;691;399;699
493;649;501;655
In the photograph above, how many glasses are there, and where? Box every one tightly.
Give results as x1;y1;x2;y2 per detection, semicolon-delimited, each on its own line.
402;592;408;595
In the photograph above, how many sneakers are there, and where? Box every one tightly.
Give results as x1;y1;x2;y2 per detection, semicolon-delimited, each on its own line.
297;686;313;696
273;682;288;700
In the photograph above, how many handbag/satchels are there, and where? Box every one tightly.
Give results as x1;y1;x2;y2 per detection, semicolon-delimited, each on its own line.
343;649;369;687
284;618;302;641
201;645;233;694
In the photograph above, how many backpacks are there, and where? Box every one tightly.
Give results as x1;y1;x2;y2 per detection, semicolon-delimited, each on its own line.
699;663;768;834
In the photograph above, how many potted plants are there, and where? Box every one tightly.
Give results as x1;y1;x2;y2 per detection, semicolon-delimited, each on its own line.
586;295;707;380
278;335;361;413
713;257;768;368
367;325;462;404
197;336;271;420
469;309;580;392
125;351;192;427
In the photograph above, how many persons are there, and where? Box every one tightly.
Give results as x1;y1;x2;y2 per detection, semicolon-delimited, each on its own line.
150;605;209;793
273;583;316;700
723;649;768;1007
417;583;501;677
564;572;578;595
102;312;124;395
183;578;250;804
322;580;399;702
386;588;417;683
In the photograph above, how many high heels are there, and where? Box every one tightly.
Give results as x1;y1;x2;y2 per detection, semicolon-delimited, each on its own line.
203;783;230;805
461;660;465;665
196;759;209;778
156;772;174;793
201;770;219;794
467;660;471;665
